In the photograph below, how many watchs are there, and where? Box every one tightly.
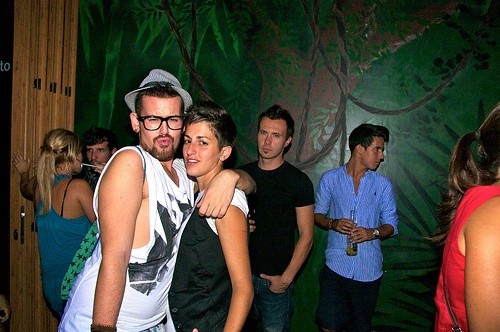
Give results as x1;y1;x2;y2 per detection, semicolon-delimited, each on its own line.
373;228;379;239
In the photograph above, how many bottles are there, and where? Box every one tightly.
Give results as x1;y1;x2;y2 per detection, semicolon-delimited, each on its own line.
346;211;359;256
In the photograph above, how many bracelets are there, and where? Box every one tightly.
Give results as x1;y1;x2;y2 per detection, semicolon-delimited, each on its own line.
90;323;117;332
329;219;335;229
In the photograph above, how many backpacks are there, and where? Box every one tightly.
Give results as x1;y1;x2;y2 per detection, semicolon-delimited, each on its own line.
61;146;146;301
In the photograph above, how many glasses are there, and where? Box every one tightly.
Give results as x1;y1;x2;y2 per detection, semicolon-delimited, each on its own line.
137;112;186;131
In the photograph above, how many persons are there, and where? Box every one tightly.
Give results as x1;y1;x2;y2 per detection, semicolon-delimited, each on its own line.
314;123;398;332
12;125;117;319
423;104;500;332
235;105;316;332
166;101;255;332
57;69;256;332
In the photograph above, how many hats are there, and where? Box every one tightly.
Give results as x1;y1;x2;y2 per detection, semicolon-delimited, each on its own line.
124;69;194;115
